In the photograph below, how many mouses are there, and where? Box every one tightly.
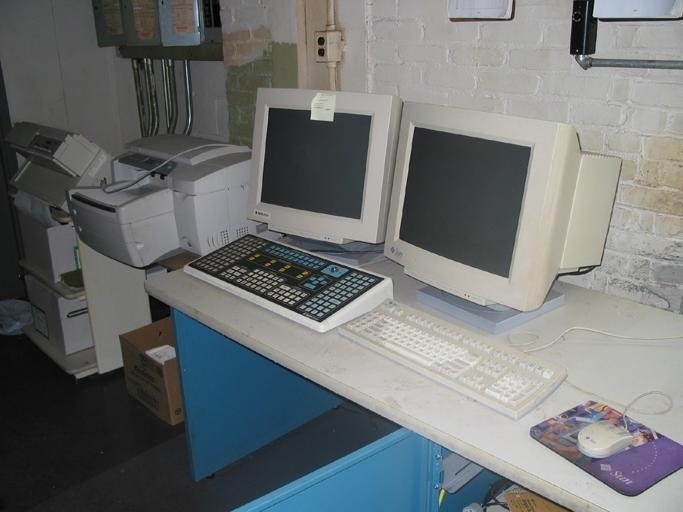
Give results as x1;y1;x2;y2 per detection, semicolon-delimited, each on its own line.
578;421;633;459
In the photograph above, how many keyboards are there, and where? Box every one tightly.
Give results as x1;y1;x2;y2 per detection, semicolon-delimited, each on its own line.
339;299;567;419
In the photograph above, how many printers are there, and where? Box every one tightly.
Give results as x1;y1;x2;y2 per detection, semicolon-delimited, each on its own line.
66;133;253;266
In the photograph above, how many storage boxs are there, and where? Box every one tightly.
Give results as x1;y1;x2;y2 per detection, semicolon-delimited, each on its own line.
118;313;184;427
23;272;94;357
17;210;81;285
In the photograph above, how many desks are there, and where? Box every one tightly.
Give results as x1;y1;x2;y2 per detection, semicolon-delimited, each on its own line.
143;235;682;511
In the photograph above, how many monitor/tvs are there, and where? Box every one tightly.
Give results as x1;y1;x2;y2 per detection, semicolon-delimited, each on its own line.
384;101;623;332
247;88;405;268
